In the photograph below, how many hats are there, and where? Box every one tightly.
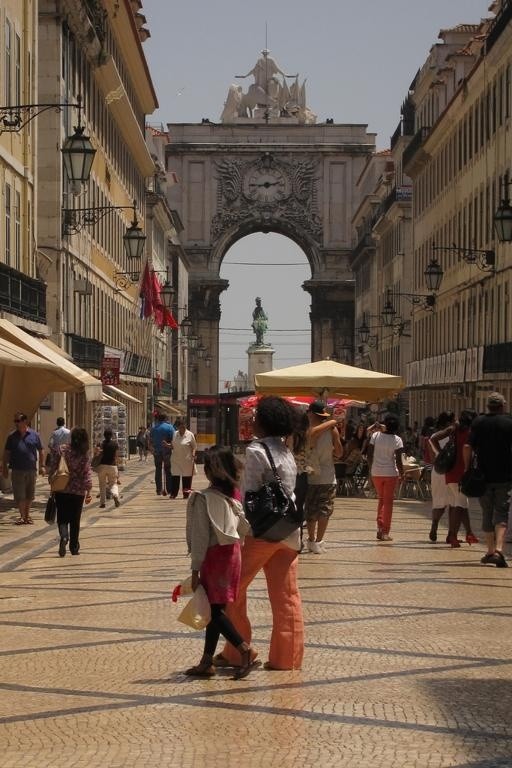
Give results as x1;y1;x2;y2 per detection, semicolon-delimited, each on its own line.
488;392;507;407
309;402;330;417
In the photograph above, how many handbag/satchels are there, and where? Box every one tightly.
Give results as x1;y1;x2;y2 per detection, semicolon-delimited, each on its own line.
435;438;456;474
45;495;56;522
45;453;56;465
49;452;70;492
91;441;104;467
245;479;300;542
461;469;485;497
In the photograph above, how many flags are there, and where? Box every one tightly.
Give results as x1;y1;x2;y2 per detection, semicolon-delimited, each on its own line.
139;264;178;329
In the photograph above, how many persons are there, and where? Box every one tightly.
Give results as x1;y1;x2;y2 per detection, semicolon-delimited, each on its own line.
211;393;305;671
235;48;298;108
2;412;45;525
47;424;92;557
135;426;147;461
47;416;71;455
286;391;512;569
161;419;197;499
147;414;175;496
183;444;263;679
95;429;121;509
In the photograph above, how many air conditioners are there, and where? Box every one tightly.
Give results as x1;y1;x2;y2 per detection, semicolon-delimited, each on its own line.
145;176;163;202
74;280;93;294
450;386;462;394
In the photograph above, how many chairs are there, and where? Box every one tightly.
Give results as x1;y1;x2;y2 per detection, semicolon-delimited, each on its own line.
334;455;434;500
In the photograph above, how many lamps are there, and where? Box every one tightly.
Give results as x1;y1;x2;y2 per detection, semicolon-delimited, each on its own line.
62;200;146;260
493;168;512;243
381;289;436;326
358;312;393;342
178;315;213;368
113;266;175;312
0;94;97;195
424;242;495;291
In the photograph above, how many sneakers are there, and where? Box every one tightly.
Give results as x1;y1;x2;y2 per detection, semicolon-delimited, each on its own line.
100;504;105;508
430;528;478;547
307;539;327;553
114;495;120;506
59;538;80;556
157;490;189;498
376;519;393;541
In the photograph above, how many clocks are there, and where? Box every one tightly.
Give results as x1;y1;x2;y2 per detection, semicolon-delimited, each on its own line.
242;161;292;202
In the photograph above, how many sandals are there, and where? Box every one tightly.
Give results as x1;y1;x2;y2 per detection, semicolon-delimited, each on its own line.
481;550;508;567
16;517;34;525
184;646;270;680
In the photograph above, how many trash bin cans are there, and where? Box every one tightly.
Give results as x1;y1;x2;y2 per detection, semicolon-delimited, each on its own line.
128;436;138;454
